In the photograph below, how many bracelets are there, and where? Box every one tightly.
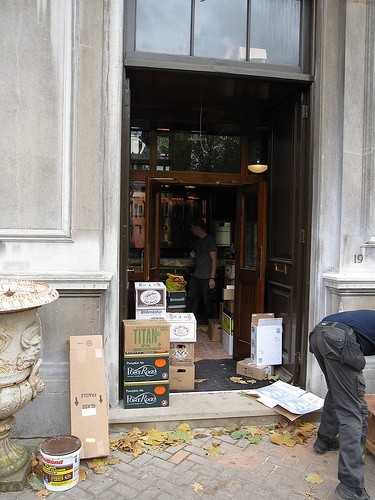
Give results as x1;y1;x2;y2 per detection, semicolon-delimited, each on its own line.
210;277;216;280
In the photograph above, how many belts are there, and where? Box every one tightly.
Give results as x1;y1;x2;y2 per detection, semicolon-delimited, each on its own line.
318;320;356;341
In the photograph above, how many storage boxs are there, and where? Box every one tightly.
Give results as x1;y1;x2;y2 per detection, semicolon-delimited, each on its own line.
68;281;374;461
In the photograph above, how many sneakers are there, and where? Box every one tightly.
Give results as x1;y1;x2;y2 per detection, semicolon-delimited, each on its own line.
335;482;373;500
313;437;340;453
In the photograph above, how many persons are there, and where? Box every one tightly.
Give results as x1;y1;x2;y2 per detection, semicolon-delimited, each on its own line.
308;309;375;500
191;215;217;323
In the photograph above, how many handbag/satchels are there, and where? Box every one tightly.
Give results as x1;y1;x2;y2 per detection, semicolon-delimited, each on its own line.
339;332;366;371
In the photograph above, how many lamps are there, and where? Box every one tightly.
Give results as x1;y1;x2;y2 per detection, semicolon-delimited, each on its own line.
247;149;270;175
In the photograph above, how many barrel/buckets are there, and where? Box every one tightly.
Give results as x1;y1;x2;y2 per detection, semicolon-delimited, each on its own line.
40;434;82;492
